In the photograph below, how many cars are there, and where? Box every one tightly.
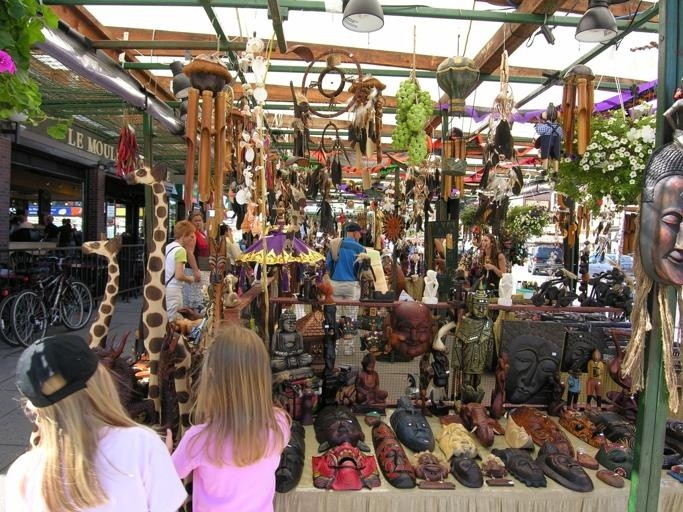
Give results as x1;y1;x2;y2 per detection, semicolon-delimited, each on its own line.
578;254;633;277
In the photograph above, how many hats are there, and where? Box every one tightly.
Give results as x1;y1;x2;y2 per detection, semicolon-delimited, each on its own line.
14;333;100;408
345;222;361;232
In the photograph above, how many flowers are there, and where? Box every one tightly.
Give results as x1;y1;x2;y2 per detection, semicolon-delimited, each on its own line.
460;201;552;265
553;105;658;217
1;0;61;126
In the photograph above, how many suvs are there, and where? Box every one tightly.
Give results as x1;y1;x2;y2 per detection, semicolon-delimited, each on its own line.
528;246;564;275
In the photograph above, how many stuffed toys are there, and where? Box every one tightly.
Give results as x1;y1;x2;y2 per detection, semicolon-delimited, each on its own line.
479;97;524;196
122;160;201;512
21;236;121;446
535;101;564;176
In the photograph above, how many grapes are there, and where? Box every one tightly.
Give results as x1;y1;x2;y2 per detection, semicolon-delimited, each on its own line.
408;133;428;165
408;104;426;131
393;111;413;151
418;92;433;115
396;79;416;109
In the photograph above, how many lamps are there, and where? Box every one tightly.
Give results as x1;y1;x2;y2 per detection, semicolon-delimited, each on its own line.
341;1;388;33
575;0;619;43
168;59;193;101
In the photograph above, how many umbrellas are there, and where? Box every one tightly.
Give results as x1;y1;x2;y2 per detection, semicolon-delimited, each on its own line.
235;202;325;297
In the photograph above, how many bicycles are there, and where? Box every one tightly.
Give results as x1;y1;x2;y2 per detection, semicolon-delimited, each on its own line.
0;256;93;346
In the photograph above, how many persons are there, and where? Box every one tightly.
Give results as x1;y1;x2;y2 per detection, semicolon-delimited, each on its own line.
183;209;209;308
474;234;504;299
354;354;390;415
42;215;59;242
640;141;683;290
165;221;196;322
384;299;434;356
585;350;604;413
357;234;392;302
397;233;429;276
9;216;33;234
452;282;494;410
423;269;441;306
59;218;77;241
325;222;368;357
213;225;241;279
167;324;289;512
270;311;313;383
2;336;190;511
566;364;583;411
492;320;567;416
